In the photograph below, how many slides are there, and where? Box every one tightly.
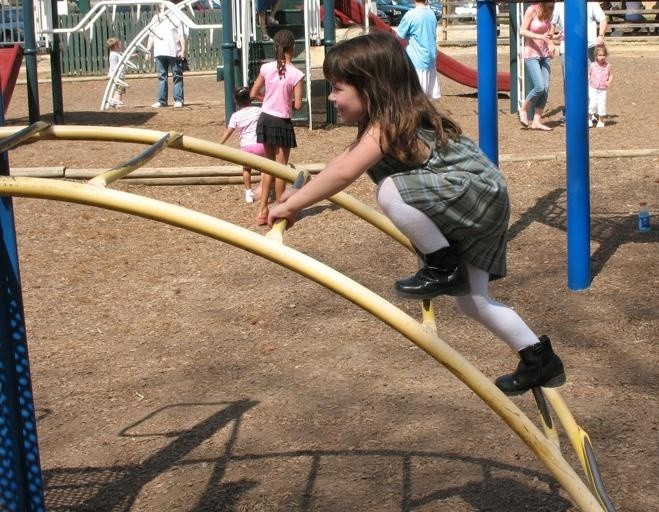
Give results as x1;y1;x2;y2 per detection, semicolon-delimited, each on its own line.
321;1;511;92
1;44;22;115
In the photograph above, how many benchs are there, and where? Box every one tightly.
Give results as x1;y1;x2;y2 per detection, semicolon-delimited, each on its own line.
593;9;659;38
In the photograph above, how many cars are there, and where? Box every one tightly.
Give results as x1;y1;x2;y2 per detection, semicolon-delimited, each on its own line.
0;5;25;48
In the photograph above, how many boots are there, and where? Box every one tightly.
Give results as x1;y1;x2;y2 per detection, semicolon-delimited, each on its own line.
492;333;565;398
393;243;472;301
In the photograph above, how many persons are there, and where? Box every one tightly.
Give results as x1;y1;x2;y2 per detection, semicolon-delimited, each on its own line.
144;3;186;108
267;25;566;397
219;87;268;204
395;0;442;100
517;1;659;131
249;30;305;226
255;0;280;41
107;37;138;108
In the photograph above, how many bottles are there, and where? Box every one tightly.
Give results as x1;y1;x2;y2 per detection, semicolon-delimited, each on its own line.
554;25;561;45
638;201;651;233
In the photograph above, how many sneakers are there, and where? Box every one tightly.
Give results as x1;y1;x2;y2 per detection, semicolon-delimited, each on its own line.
244;188;256;204
516;107;529;130
531;121;554;131
173;98;185;109
255;214;268;226
596;119;605;128
588;119;594;128
150;99;168;109
